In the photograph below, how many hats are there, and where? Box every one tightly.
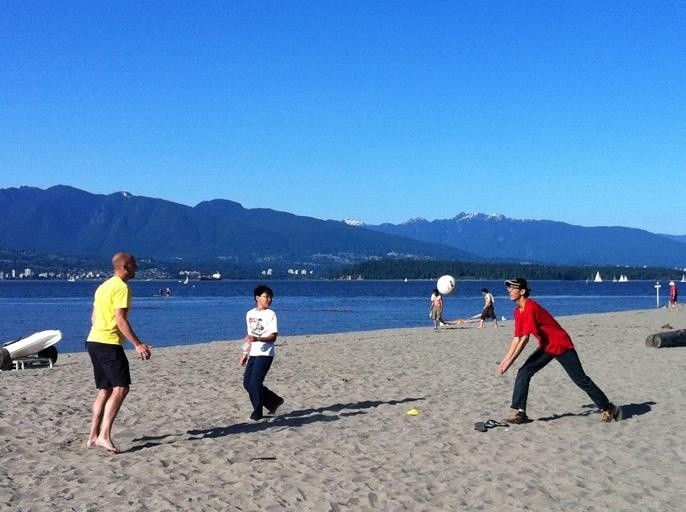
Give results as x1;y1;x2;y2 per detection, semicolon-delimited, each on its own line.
504;277;527;286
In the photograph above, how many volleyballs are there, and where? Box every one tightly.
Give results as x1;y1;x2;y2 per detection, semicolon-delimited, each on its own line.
437;275;455;295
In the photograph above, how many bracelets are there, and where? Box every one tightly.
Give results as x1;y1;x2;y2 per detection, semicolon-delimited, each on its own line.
258;337;266;343
242;351;248;355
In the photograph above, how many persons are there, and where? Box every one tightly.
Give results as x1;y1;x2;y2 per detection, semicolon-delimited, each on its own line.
475;288;499;330
428;289;449;328
665;283;679;312
430;293;442;331
81;249;152;453
495;277;620;425
236;281;284;422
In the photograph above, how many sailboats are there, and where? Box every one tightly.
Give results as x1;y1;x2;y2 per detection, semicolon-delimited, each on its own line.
593;271;602;282
612;274;628;283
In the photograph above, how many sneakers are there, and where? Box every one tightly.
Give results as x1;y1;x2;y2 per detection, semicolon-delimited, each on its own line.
599;403;618;423
268;397;284;414
505;411;528;424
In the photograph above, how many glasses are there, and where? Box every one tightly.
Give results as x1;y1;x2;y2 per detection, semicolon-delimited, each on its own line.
507;287;520;291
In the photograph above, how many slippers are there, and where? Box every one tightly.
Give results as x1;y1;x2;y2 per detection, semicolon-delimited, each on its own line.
474;420;508;432
